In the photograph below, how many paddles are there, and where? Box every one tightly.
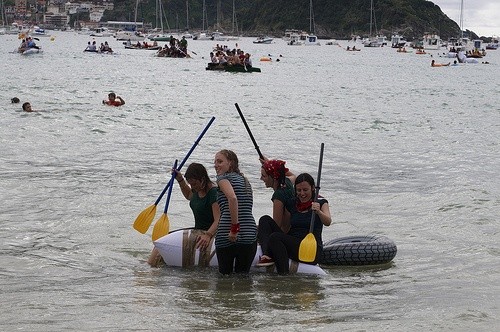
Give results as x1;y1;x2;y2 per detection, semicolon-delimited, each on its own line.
296;142;324;262
234;103;264;159
133;116;215;234
152;159;178;243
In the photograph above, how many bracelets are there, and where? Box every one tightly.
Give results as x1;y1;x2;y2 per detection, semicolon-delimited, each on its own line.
231;222;240;234
178;177;184;182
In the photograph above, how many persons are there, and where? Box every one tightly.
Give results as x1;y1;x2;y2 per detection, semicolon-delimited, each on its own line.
259;173;331;276
83;41;109;51
102;92;125;107
215;149;257;274
126;35;188;57
17;37;41;54
287;40;487;67
171;163;220;252
210;44;252;71
255;156;296;267
11;97;31;112
268;53;283;61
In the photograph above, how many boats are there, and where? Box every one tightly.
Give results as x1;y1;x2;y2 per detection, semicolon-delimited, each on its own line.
282;0;500;67
153;227;326;276
0;22;51;37
205;63;261;72
22;48;38;54
84;49;98;53
253;34;273;44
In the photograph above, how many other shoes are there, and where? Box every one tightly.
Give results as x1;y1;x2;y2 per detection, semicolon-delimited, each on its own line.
256;255;275;267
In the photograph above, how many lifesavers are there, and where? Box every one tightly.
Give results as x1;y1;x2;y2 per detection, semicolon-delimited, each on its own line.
323;233;398;265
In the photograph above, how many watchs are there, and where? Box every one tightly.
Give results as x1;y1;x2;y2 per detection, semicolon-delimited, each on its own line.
206;232;212;238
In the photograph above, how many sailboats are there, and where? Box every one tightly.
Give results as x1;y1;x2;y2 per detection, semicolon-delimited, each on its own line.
78;0;242;50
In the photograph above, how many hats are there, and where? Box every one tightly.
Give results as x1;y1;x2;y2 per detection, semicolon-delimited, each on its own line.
262;159;290;180
